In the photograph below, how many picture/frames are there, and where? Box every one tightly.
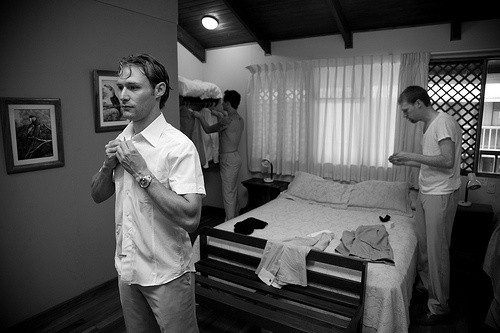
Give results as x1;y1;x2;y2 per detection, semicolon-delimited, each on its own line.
92;68;131;133
0;97;65;175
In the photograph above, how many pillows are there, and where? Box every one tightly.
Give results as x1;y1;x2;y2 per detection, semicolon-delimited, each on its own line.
280;171;353;210
348;180;414;218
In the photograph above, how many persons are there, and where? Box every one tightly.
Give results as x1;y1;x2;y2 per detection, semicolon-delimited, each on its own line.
91;54;205;333
388;85;461;326
193;89;244;222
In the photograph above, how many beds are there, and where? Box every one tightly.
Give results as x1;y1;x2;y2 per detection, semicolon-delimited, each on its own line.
192;177;419;333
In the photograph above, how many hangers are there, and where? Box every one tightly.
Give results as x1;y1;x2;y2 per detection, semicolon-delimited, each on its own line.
181;101;216;109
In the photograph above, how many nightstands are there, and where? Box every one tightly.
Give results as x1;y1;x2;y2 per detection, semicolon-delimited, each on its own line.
449;203;494;271
239;178;290;214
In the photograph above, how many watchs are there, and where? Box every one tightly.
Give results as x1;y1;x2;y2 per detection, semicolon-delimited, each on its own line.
138;174;155;188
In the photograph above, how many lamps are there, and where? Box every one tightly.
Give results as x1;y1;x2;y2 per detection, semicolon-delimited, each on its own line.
260;154;274;182
457;172;481;207
201;15;219;30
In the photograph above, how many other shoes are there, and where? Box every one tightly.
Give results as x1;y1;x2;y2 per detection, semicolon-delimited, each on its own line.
411;310;449;326
